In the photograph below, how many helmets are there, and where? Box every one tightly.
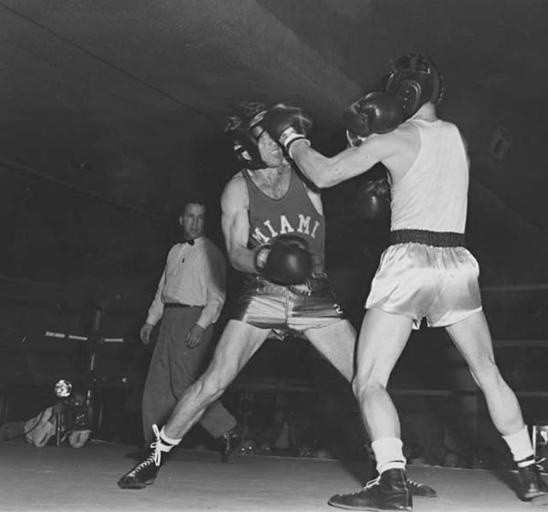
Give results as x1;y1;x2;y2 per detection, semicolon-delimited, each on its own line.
229;109;268;171
385;51;444;123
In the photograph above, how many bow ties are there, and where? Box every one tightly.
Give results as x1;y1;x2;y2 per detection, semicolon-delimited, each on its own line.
179;237;194;245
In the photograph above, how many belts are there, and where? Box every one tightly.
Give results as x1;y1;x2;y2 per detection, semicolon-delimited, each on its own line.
164;303;204;309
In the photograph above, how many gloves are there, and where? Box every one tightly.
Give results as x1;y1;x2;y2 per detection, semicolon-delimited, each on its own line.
342;91;403;137
260;107;313;159
253;242;313;286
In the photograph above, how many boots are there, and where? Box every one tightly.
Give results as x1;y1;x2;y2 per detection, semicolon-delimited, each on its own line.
118;423;183;489
363;439;437;498
327;460;412;512
514;454;546;501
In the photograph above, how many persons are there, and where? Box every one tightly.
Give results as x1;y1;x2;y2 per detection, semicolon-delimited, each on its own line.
136;195;247;466
196;406;521;471
258;51;548;512
114;97;439;496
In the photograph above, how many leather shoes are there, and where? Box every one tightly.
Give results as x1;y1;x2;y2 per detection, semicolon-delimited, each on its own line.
222;424;244;463
125;447;153;459
